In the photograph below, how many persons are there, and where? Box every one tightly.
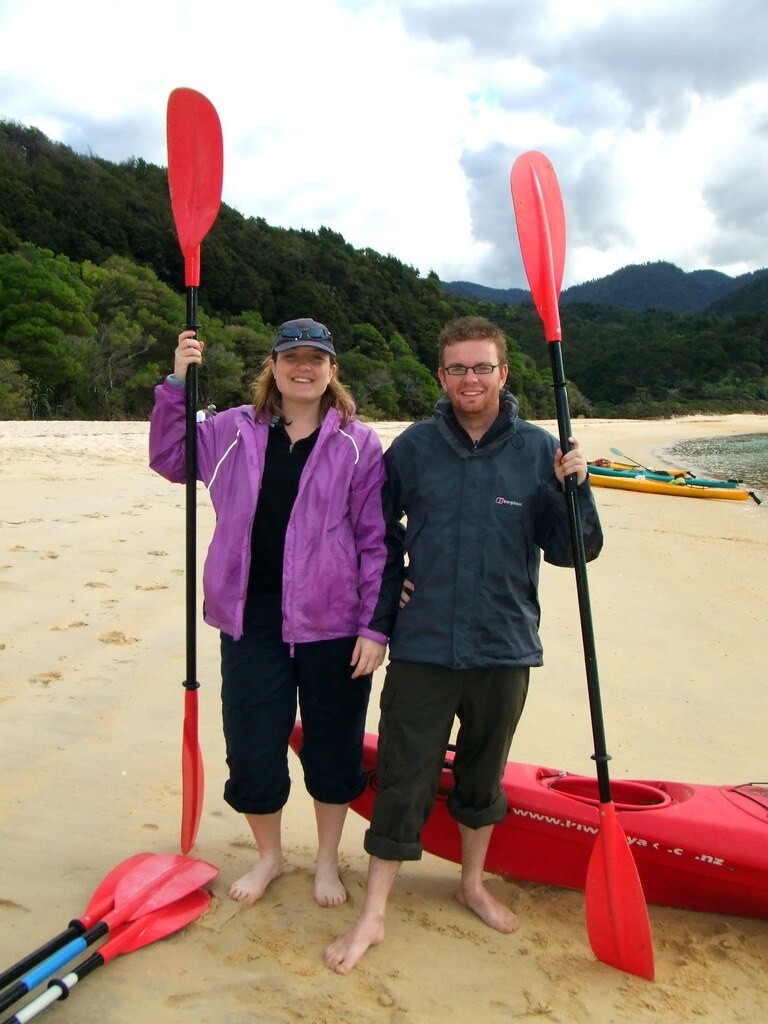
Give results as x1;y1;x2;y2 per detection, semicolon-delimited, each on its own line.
150;319;406;907
325;319;603;974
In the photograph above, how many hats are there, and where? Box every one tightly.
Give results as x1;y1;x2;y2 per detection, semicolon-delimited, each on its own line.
271;317;335;355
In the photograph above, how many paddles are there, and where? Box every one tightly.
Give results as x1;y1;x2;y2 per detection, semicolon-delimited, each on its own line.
609;447;651;472
159;82;232;862
506;144;667;985
0;850;224;1024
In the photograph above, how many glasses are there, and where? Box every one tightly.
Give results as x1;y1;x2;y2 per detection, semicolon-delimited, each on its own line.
274;326;333;343
444;364;500;375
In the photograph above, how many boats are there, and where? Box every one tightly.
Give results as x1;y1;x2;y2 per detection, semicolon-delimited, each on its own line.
287;719;767;921
581;458;761;506
